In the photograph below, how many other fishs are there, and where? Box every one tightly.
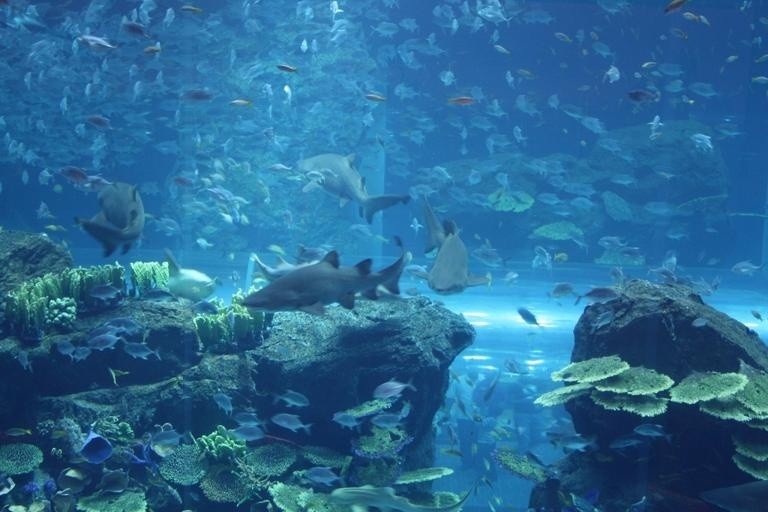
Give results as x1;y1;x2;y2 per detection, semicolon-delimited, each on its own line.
2;0;768;512
